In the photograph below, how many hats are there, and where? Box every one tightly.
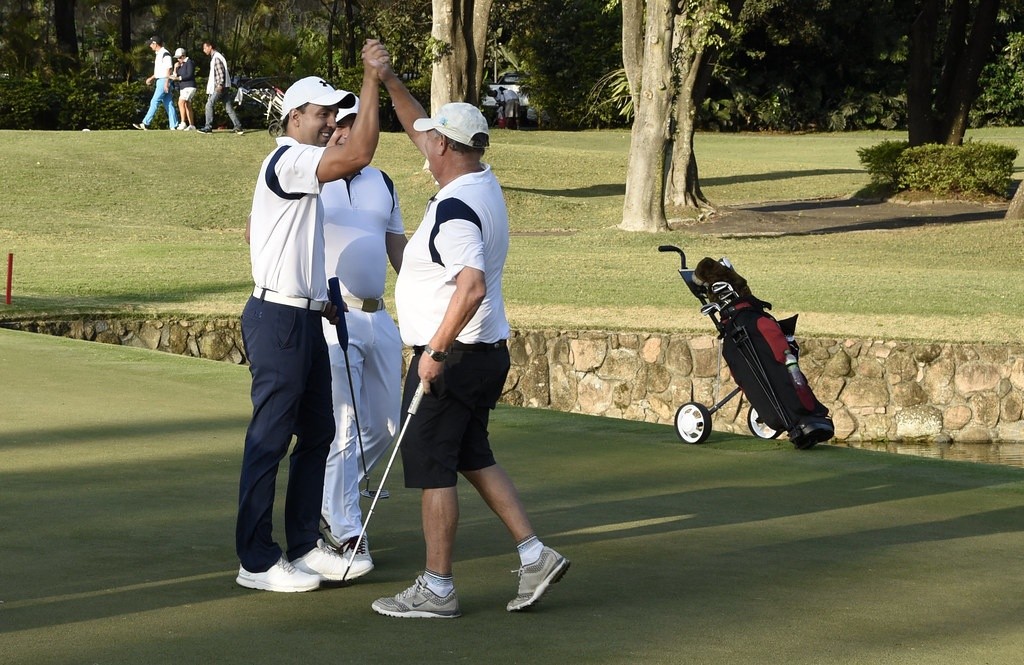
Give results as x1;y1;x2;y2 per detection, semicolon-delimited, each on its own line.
172;48;186;58
145;35;162;44
280;76;356;121
334;94;359;124
413;102;490;149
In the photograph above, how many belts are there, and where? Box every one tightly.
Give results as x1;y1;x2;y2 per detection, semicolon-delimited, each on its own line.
252;285;332;314
412;339;507;357
341;296;386;313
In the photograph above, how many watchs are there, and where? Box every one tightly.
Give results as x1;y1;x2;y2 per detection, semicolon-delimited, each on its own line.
424;345;448;362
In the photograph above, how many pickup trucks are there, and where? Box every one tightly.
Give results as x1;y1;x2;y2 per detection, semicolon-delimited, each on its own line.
481;67;529;108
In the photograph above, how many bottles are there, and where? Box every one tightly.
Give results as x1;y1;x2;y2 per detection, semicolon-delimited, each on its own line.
785;350;805;387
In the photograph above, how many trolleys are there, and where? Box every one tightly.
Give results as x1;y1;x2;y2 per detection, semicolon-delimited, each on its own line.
658;245;836;450
239;80;285;137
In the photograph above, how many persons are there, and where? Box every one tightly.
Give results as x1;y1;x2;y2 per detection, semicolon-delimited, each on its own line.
486;87;520;131
362;39;571;618
197;41;244;135
169;48;197;131
133;36;179;130
233;40;390;593
322;87;409;563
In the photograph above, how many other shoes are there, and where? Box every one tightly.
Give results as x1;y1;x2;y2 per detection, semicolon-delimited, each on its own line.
169;125;176;130
197;126;212;134
184;125;196;131
133;123;145;130
177;123;187;130
232;127;244;135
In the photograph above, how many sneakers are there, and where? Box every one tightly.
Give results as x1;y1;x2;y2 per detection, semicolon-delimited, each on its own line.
371;575;461;619
506;546;570;612
290;539;374;582
339;536;374;581
235;556;320;593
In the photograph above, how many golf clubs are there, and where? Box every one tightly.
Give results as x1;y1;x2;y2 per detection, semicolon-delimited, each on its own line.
700;282;740;314
340;382;425;586
327;276;391;500
